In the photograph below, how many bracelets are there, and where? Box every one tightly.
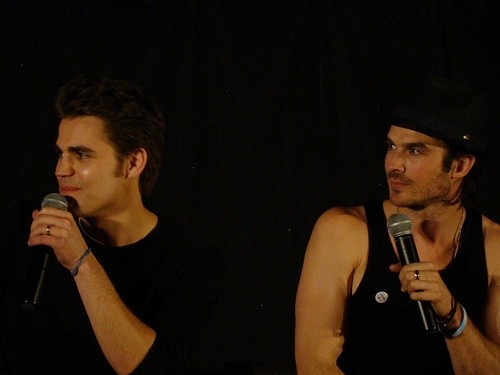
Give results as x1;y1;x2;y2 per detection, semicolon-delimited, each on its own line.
431;294;458;327
71;247;91;276
438;303;467;339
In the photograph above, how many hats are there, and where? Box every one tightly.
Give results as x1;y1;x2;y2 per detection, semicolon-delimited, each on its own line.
386;76;490;160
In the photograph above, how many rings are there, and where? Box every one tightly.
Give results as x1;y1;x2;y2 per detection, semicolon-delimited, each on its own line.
46;223;51;235
415;270;418;279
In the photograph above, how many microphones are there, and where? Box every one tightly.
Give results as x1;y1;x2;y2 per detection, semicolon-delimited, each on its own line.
25;193;68;304
387;213;436;330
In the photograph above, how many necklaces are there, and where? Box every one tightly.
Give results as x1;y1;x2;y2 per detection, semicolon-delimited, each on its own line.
396;203;465;259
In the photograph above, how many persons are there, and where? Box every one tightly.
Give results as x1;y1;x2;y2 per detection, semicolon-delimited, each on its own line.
0;75;224;375
295;86;500;375
